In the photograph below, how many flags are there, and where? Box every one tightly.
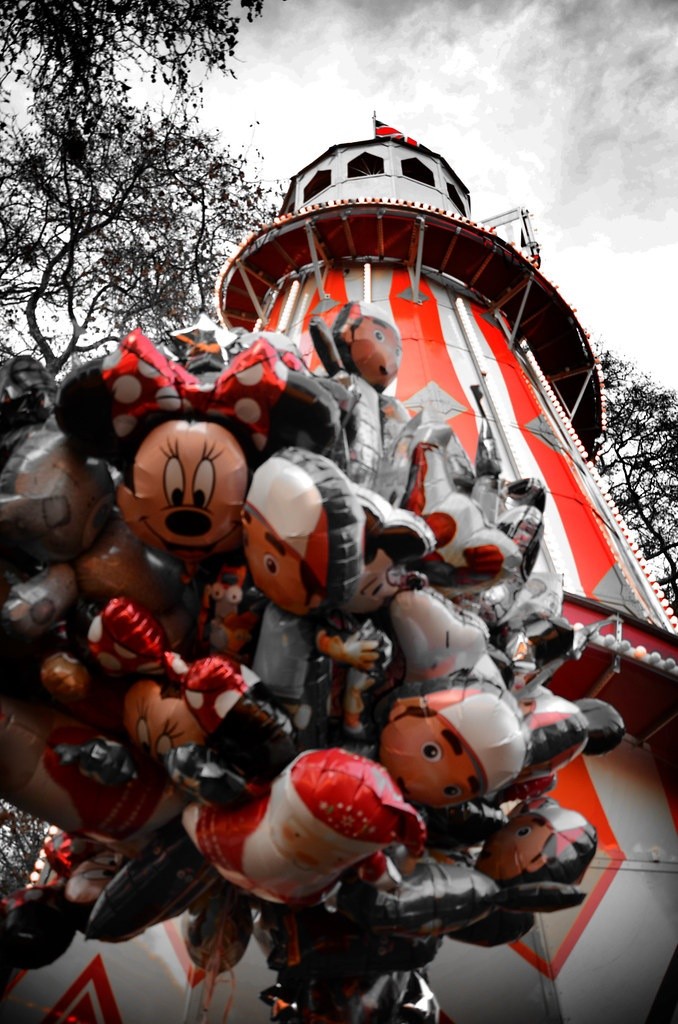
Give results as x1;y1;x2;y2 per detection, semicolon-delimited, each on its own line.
376;116;420;146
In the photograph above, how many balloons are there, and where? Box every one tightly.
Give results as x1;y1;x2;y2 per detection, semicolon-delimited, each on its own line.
0;301;624;1024
